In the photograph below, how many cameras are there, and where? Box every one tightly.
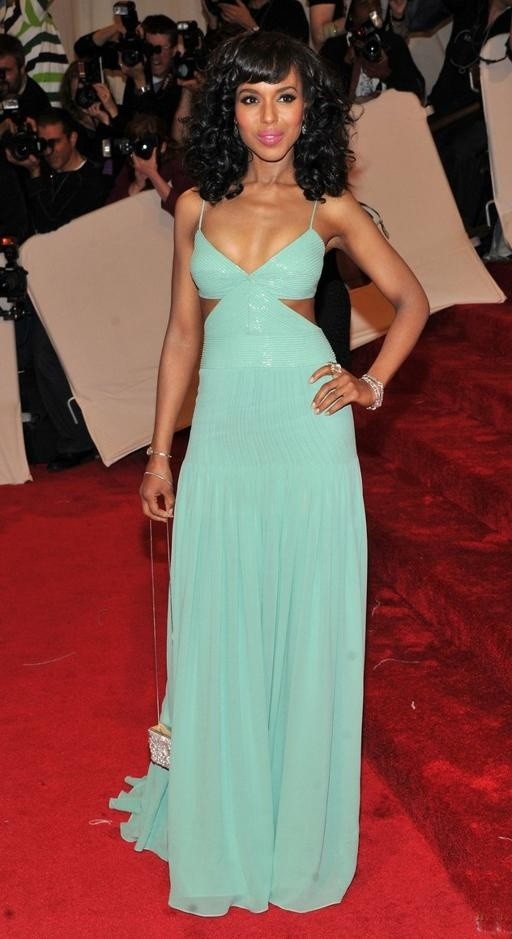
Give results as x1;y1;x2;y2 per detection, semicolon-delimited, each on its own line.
112;1;154;67
76;84;101;107
351;11;385;62
0;69;23;120
100;136;163;160
10;125;46;162
172;20;206;79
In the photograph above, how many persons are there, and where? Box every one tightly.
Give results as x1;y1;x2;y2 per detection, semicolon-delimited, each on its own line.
110;26;429;918
0;1;512;470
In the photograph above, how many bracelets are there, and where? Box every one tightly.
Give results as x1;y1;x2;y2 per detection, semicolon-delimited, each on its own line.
140;470;177;490
